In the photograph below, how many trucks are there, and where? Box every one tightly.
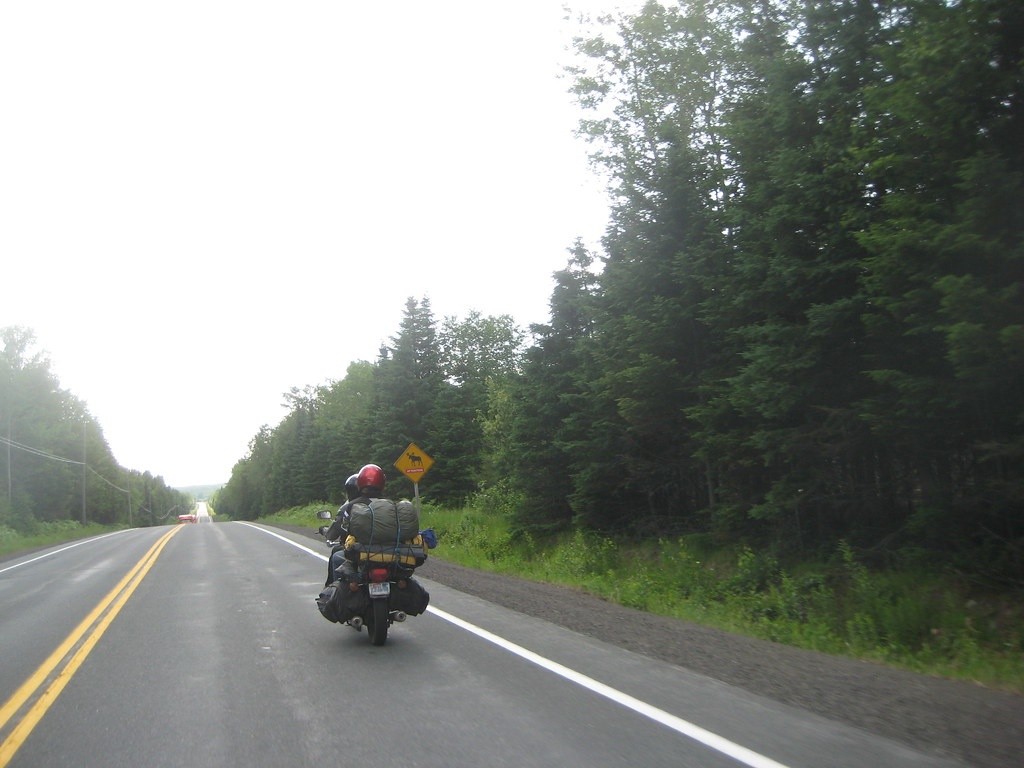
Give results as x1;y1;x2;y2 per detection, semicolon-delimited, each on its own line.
179;514;195;524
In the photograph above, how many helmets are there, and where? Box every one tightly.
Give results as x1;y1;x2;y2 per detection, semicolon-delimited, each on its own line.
356;463;385;492
345;473;359;490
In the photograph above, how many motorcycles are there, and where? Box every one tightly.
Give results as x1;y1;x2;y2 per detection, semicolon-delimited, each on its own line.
315;510;406;646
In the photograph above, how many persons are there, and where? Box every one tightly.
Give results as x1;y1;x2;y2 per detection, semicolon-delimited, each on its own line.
315;463;386;589
